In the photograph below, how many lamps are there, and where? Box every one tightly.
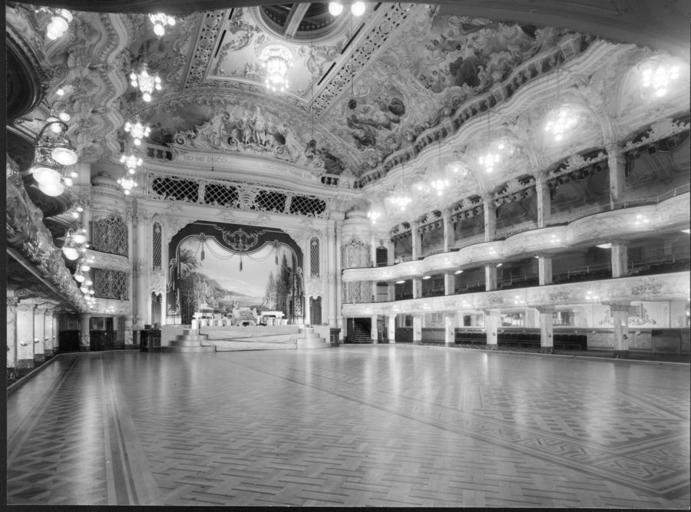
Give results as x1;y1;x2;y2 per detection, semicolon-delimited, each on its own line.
327;0;370;18
